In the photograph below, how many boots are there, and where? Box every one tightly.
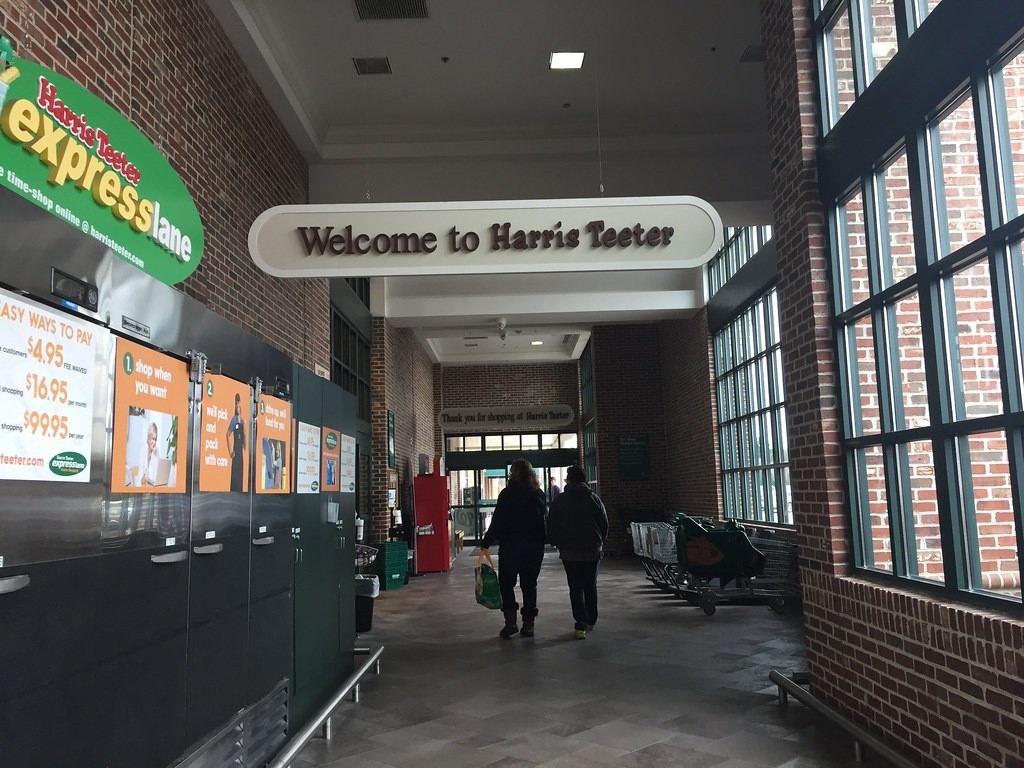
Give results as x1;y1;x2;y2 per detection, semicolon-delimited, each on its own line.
521;606;538;637
499;603;518;637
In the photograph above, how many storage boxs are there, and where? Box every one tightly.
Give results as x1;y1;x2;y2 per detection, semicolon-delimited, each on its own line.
369;541;408;591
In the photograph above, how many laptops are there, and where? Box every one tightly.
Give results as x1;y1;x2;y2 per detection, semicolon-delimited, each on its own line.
148;459;172;486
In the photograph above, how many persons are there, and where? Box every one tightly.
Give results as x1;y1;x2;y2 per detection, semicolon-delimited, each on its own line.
226;393;246;492
130;422;163;487
547;465;610;639
481;458;546;638
271;440;282;489
543;477;561;501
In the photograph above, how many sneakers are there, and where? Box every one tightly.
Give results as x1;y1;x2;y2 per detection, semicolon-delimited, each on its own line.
575;628;587;640
589;623;595;632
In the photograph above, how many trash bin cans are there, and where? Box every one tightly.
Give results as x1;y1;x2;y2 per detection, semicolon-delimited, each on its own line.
355;574;379;633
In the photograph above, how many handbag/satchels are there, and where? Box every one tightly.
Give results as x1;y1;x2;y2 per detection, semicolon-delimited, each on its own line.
474;548;503;609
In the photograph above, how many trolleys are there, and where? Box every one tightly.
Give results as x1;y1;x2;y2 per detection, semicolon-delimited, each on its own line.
629;512;792;617
